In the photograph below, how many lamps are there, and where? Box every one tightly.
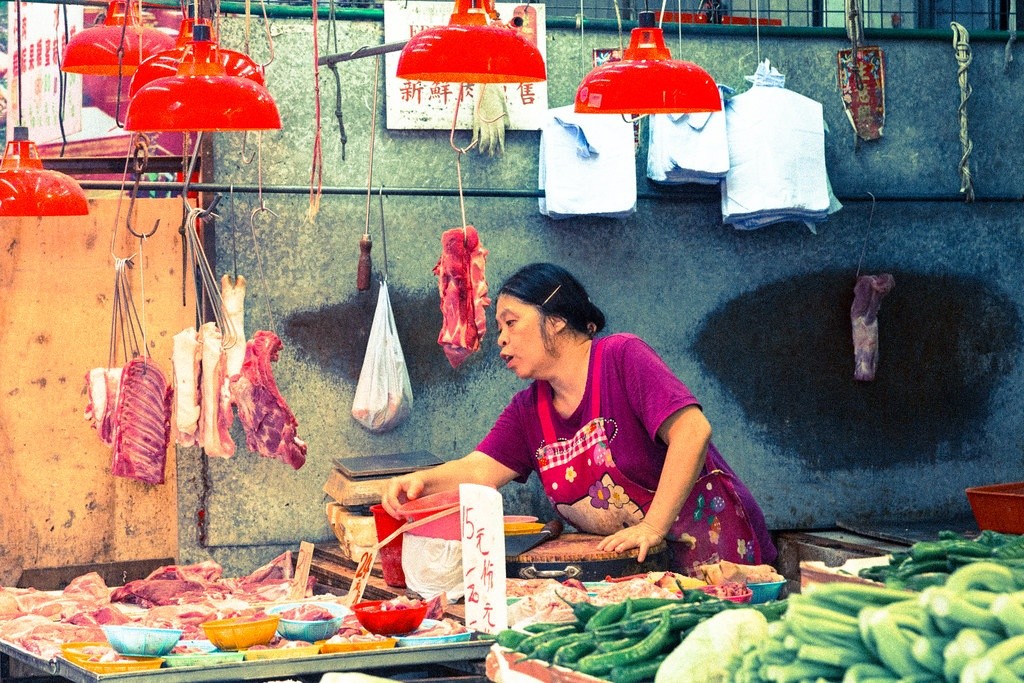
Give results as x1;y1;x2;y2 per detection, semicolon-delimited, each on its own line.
60;0;283;130
0;0;89;216
396;0;546;83
575;0;721;113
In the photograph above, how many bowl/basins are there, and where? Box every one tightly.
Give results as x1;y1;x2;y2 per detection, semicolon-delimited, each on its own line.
502;515;539;523
350;600;430;636
504;523;546;535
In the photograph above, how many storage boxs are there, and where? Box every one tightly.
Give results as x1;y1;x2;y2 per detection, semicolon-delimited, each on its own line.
485;643;616;683
801;556;888;598
326;500;378;563
778;526;910;582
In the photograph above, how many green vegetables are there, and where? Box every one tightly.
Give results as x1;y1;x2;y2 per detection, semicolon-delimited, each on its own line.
717;562;1024;683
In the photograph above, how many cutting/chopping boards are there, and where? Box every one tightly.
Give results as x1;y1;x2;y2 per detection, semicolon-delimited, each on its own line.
506;532;669;581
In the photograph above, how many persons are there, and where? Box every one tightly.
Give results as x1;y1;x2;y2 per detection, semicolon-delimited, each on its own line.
383;263;785;573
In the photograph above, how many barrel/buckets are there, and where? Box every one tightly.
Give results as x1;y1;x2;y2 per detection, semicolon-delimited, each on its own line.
369;502;407;588
396;488;462;542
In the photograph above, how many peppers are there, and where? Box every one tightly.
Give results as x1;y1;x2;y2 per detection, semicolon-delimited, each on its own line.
835;529;1024;593
477;578;790;683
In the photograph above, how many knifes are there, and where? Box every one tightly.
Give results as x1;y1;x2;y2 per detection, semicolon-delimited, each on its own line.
504;520;563;558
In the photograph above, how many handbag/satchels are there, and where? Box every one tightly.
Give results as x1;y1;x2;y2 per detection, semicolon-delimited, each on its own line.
352;281;414;432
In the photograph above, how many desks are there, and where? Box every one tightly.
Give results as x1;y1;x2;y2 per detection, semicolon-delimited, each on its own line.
297;544;465;625
